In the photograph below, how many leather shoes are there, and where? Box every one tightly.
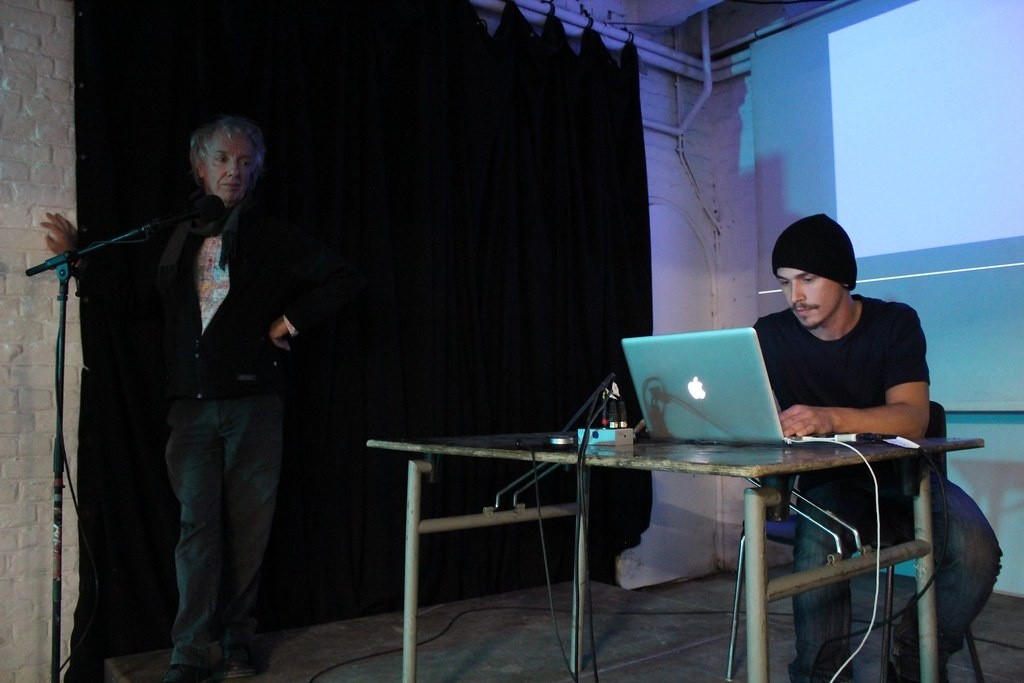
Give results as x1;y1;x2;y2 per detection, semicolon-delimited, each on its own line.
157;661;212;683
219;628;257;679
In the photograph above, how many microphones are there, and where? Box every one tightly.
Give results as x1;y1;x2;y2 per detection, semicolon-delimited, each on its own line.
151;195;226;230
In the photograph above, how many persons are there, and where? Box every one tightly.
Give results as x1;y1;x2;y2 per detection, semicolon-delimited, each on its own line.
632;214;1003;683
42;117;367;683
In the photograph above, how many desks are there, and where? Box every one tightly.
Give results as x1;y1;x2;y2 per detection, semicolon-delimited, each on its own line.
366;437;989;682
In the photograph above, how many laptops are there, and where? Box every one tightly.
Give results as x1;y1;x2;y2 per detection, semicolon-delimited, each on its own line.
623;326;880;445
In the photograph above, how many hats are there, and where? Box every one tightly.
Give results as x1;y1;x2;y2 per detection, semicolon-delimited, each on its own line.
771;213;858;288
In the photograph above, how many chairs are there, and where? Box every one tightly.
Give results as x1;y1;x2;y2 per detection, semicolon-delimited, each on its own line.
726;401;986;681
